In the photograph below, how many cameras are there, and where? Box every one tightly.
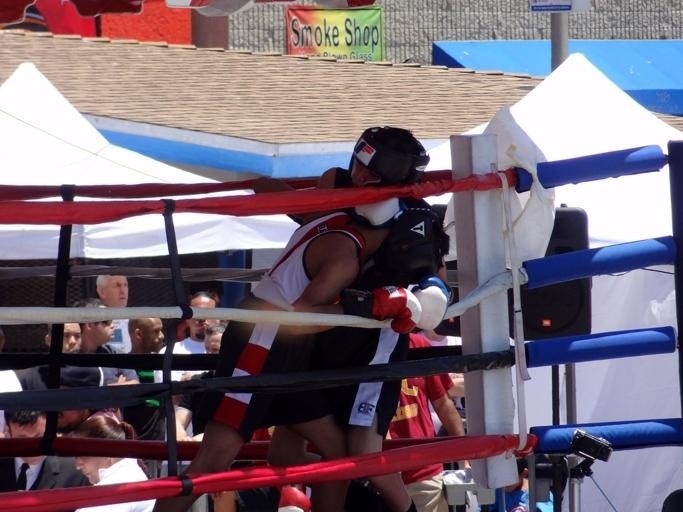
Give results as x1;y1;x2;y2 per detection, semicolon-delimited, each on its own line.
567;429;613;464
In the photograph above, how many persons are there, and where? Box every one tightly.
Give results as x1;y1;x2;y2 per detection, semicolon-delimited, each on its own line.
253;125;454;510
153;197;445;511
1;274;531;512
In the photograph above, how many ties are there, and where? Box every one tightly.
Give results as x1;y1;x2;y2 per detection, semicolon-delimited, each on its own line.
16;462;30;492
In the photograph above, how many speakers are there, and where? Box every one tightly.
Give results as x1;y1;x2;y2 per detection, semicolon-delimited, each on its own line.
509;203;593;341
429;203;460;336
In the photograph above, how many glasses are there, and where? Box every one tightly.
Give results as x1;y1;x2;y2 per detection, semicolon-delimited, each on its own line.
103;320;113;326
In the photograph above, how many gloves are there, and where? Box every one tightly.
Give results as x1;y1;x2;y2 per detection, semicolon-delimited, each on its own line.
339;285;423;335
409;275;451;332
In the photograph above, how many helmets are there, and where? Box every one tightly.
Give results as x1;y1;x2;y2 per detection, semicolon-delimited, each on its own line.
370;208;450;285
348;124;429;188
